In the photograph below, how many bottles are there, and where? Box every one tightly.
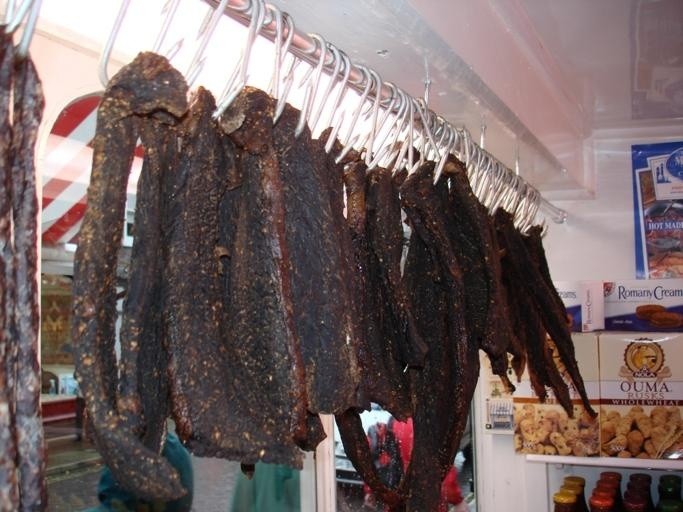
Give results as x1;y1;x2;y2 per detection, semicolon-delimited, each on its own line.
553;493;577;512
623;491;645;511
590;495;614;512
557;470;683;499
657;495;682;512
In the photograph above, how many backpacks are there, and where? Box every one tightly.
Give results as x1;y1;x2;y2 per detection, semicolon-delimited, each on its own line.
366;422;405;504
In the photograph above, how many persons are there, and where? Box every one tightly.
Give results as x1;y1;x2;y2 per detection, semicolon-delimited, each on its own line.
365;396;471;512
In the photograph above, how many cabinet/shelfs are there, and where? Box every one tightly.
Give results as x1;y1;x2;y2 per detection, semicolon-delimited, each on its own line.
472;351;549;512
527;451;681;471
